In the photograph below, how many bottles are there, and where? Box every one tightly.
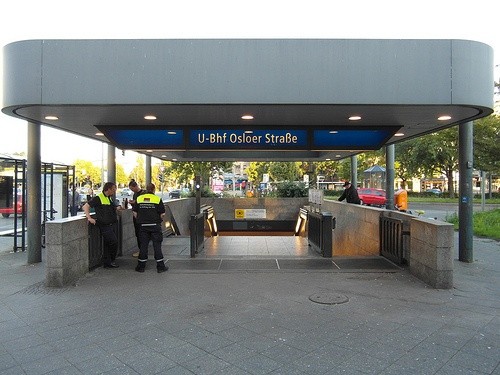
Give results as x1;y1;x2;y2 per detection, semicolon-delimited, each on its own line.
125;198;128;209
122;198;125;209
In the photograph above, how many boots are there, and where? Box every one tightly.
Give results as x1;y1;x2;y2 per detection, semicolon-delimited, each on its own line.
135;261;146;272
156;260;169;273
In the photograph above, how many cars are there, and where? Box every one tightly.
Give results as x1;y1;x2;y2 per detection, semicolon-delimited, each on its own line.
169;189;188;199
120;188;134;196
357;187;395;208
0;192;28;218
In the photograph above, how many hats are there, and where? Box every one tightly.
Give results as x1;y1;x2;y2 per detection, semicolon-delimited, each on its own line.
343;182;351;187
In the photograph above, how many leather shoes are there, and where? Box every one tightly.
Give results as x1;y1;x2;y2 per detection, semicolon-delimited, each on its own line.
104;261;120;268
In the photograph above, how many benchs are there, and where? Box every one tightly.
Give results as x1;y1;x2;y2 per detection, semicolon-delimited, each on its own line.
0;227;28;237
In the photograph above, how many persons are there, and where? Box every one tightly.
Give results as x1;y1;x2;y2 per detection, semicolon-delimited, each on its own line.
83;182;123;268
338;182;360;205
129;179;146;257
135;183;168;273
